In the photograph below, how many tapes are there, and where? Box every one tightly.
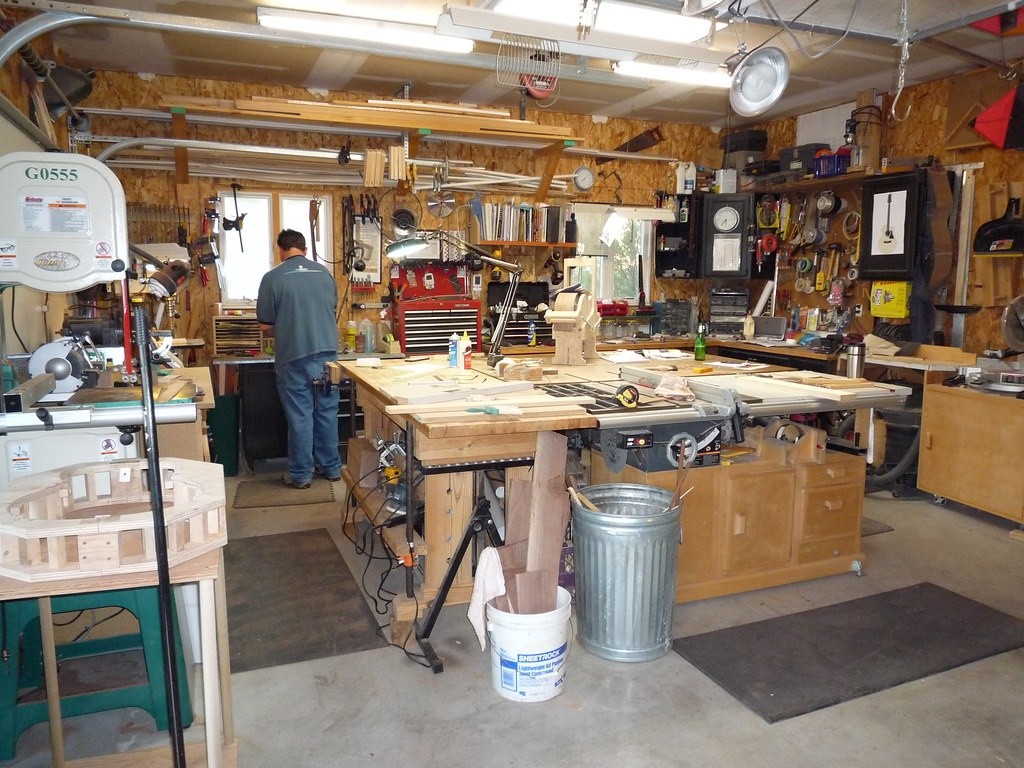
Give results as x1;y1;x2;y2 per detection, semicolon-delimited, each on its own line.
817;196;831;212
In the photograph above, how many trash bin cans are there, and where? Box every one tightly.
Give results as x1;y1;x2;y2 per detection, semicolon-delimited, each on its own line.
570;484;682;664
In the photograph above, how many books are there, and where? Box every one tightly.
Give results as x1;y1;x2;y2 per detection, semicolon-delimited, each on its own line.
471;199;547;242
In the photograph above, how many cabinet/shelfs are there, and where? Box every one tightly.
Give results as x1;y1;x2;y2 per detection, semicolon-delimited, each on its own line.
916;387;1024;524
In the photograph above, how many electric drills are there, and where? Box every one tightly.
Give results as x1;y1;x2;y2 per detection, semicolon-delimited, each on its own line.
756;234;776;273
487;250;502;280
544;251;564;285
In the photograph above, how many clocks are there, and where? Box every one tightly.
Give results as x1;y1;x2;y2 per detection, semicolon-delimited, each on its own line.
573;166;595;191
714;207;739;231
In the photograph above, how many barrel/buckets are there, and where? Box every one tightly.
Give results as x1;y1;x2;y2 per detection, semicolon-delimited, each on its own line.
486;585;571;703
569;481;682;663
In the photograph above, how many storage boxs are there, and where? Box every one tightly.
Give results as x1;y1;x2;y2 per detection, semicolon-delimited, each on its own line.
715;169;737;193
742;161;779;177
719;130;768;154
777;143;832;177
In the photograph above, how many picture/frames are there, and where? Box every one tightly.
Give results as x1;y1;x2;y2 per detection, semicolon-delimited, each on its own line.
858;172;922;281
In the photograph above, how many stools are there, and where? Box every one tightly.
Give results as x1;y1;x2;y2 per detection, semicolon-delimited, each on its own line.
0;585;194;760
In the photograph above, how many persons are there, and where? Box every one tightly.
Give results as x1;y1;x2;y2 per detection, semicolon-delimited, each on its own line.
256;229;342;489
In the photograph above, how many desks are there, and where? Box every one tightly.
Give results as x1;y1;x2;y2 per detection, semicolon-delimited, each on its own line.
156;339;205;368
208;334;716;475
720;336;973;500
0;366;217;661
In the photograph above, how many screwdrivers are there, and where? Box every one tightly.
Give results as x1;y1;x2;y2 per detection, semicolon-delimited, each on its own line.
354;274;374;299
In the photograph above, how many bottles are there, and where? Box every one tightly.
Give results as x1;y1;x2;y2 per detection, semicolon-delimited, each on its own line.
743;314;754;340
345;318;376;355
528;320;536;347
675;161;684;194
459;331;472;369
684;180;695;194
597;319;638;341
449;332;460;367
685;161;695;190
656;195;688;222
694;322;706;361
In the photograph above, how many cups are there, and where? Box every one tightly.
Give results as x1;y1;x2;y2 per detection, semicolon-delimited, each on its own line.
814;155;835;177
835;153;851;176
658;235;665;250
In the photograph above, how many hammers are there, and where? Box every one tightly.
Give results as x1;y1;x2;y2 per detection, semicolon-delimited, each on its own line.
826;244;841;281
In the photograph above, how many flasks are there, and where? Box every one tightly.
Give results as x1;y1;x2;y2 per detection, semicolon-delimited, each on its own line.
846;342;866;379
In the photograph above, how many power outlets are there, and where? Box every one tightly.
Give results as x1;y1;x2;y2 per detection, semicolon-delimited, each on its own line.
509;273;519;282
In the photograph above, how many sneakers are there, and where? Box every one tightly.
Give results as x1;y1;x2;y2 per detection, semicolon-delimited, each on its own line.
280;472;310;489
314;464;341;480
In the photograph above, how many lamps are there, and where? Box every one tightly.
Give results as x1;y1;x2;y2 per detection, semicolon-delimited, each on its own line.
612;60;734;89
386;225;525;368
724;46;791;117
256;7;477;54
129;242;190;298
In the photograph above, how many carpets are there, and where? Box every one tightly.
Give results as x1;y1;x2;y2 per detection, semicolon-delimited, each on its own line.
222;528;387;675
670;582;1024;725
861;515;895;538
232;476;336;509
